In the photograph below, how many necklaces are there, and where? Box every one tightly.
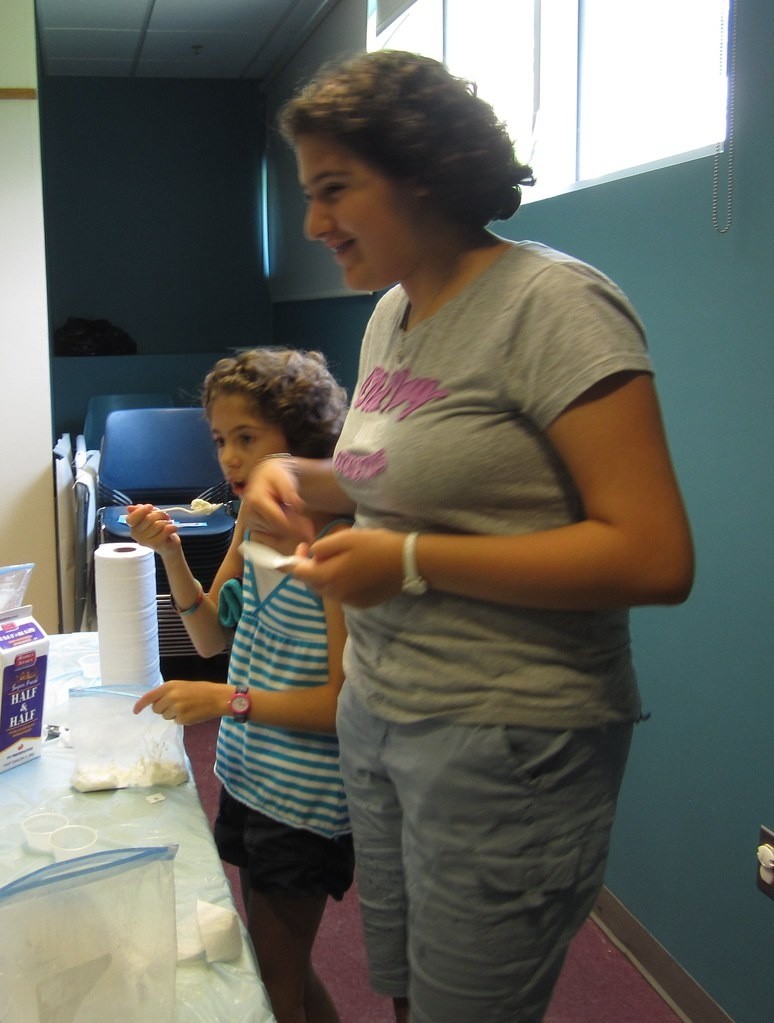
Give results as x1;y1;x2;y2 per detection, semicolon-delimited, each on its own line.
397;263;457;362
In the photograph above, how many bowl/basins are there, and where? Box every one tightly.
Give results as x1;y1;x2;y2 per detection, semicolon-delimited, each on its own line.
49;825;98;863
20;812;70;850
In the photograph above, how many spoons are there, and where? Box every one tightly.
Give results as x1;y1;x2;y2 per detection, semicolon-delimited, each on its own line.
159;503;224;517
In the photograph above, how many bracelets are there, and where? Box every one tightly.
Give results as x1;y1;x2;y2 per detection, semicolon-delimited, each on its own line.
170;579;203;616
257;453;300;481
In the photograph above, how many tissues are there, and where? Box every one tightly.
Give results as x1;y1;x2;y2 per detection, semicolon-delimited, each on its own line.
93;541;161;687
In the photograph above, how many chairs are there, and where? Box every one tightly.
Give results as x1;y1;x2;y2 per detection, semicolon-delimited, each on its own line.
73;392;238;659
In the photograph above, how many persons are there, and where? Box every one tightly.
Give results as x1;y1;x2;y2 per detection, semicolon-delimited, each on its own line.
240;50;696;1023
127;348;355;1023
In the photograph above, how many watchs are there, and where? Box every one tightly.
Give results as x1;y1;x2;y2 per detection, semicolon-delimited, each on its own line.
403;533;426;596
228;685;249;723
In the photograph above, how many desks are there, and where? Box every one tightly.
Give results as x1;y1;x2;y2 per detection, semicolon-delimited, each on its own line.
0;627;277;1023
